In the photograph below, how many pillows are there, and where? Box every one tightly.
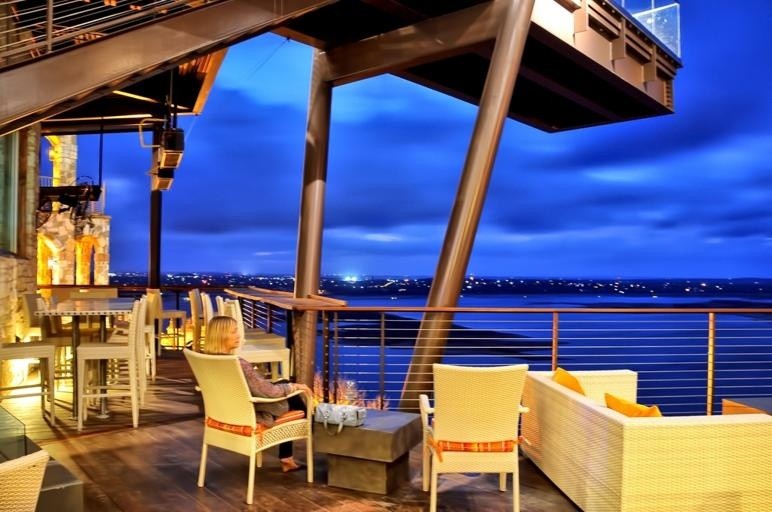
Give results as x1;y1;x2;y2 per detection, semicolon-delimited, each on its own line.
551;366;585;397
601;390;661;418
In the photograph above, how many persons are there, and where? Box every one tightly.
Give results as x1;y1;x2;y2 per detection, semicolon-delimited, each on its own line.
205;316;317;471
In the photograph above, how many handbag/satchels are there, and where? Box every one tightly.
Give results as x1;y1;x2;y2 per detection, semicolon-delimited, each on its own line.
315;403;366;435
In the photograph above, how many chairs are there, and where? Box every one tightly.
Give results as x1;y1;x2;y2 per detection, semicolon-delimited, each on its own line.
216;295;267;337
188;290;202;353
76;301;141;432
145;287;188;356
227;299;286;346
114;293;156;383
106;296;147;409
199;291;214;330
21;291;67;337
0;451;50;512
0;343;56;427
49;295;93;378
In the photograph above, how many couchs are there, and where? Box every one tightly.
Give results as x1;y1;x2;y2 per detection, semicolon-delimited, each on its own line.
521;369;772;512
420;363;532;512
181;348;314;504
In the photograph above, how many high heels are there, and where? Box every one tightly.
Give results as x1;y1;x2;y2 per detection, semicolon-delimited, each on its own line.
280;461;300;473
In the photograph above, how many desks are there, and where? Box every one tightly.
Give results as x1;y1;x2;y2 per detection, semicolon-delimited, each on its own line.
260;295;346;347
0;434;86;512
34;297;135;420
314;410;422;495
224;286;292;328
